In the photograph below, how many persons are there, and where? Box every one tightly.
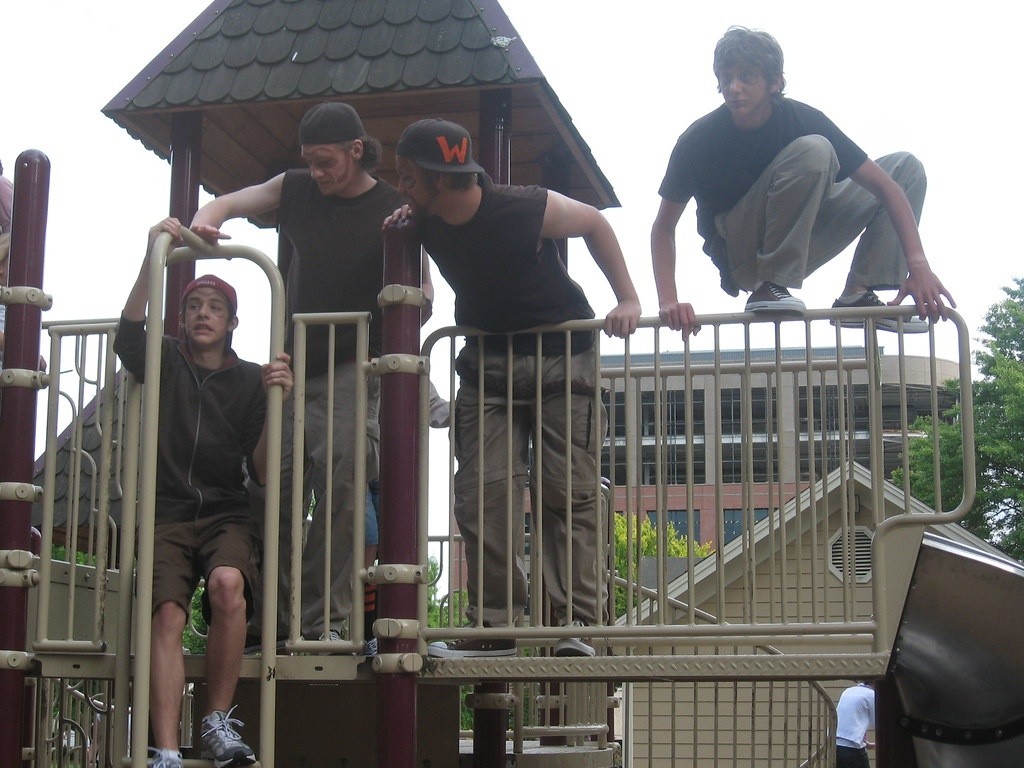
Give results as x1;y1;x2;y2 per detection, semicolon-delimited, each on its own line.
113;217;293;768
188;101;434;656
353;381;451;656
650;30;957;334
380;117;643;658
0;160;47;372
827;677;876;768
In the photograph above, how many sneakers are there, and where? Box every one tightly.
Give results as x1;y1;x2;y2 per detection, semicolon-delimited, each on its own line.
553;618;597;657
319;631;350;656
829;292;928;333
364;637;378;655
200;704;256;768
427;622;517;658
147;747;182;768
745;281;805;314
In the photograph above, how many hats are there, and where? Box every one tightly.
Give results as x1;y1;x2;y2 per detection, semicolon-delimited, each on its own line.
180;274;237;311
395;117;486;174
297;99;366;144
